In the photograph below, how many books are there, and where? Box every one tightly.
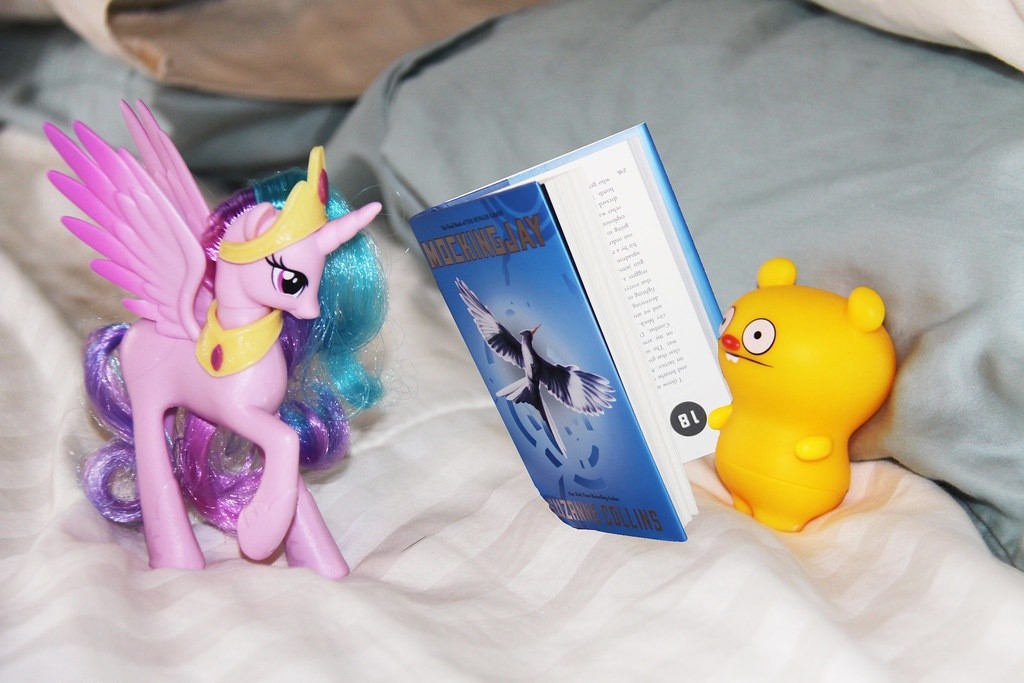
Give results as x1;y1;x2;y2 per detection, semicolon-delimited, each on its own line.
409;123;732;544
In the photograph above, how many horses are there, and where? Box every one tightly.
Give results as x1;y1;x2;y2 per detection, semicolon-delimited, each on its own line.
114;138;401;580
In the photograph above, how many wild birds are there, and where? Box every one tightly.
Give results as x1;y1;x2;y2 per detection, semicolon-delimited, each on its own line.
454;275;621;461
